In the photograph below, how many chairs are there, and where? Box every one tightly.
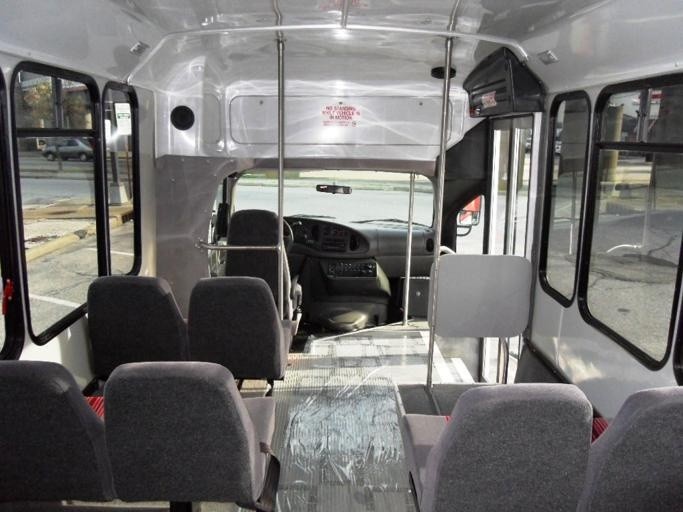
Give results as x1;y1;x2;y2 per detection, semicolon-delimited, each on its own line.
223;210;287;315
189;274;294;399
80;272;188;391
0;358;112;511
402;384;682;512
101;359;280;509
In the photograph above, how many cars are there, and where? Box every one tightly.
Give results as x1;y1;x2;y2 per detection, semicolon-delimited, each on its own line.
42;138;93;161
